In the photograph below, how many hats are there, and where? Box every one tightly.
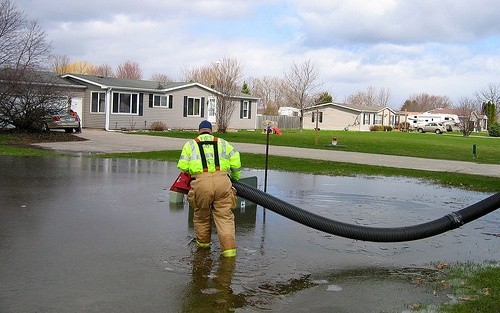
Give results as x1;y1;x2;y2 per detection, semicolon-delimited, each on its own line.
199;121;212;131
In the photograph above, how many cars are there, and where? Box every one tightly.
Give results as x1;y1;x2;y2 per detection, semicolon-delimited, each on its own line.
27;107;79;133
417;121;447;134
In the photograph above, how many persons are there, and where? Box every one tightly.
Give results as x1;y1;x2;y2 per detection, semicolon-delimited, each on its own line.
177;120;243;257
74;112;82;133
70;110;80;133
